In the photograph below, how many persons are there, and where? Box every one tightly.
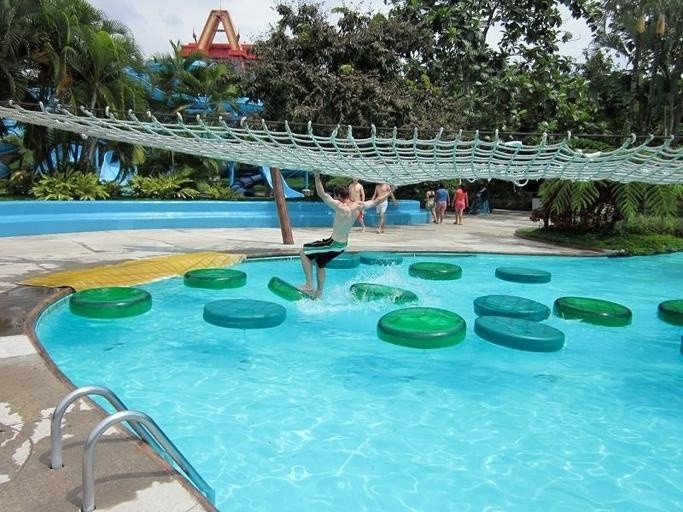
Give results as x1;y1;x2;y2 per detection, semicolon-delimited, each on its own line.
434;184;450;224
348;178;367;234
371;182;397;234
470;183;489;210
424;187;437;222
451;184;469;224
296;173;398;298
